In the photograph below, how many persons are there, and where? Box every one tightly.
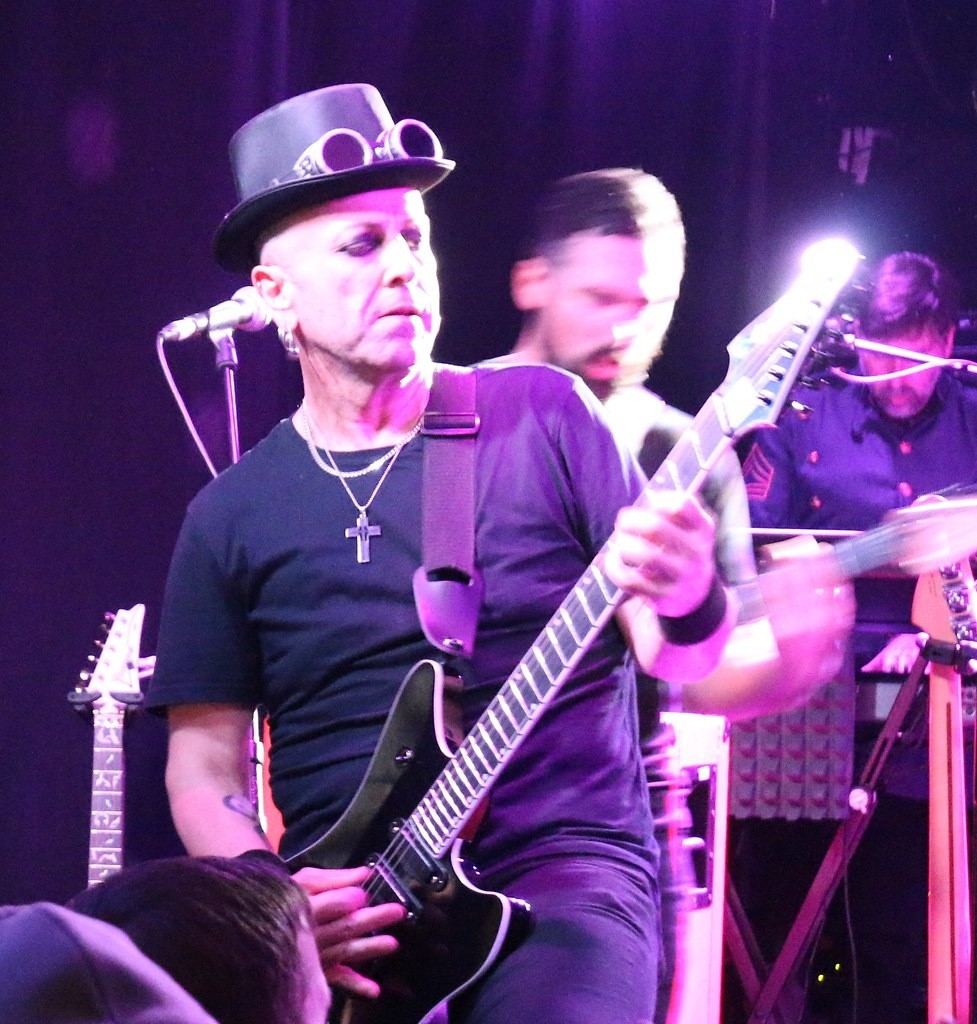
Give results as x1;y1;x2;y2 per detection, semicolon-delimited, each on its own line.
740;251;977;674
59;857;333;1024
472;168;855;1024
151;84;738;1024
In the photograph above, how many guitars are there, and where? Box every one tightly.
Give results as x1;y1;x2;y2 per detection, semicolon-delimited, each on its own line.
727;492;976;627
281;229;871;1024
903;490;977;1024
65;602;146;888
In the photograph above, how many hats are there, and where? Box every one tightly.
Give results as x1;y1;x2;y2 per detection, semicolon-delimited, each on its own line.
215;83;456;274
0;902;219;1024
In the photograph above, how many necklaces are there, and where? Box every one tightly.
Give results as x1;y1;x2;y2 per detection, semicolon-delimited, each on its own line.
303;399;422;561
299;404;423;477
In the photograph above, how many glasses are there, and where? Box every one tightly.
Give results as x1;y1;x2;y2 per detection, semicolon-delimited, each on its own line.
268;118;443;187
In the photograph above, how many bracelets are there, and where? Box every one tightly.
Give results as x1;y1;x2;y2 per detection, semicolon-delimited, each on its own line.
658;575;726;647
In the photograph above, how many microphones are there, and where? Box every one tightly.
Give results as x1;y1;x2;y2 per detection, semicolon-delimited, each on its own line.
154;283;276;343
